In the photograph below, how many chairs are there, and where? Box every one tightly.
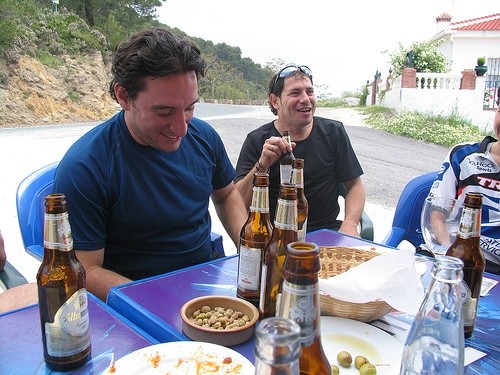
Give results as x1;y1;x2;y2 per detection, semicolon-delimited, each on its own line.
381;172;438;247
16;160;227;264
339;183;373;240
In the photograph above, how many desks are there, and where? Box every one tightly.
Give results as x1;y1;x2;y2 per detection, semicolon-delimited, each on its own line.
106;228;500;375
0;288;162;375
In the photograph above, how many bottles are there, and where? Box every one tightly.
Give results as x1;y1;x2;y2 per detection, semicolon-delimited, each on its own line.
253;317;303;375
36;193;92;372
279;129;298;185
444;192;486;339
258;184;299;319
268;242;331;375
235;172;273;308
399;254;466;375
289;158;309;242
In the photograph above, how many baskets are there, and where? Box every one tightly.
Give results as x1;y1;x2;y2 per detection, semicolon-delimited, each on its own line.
318;245;394;322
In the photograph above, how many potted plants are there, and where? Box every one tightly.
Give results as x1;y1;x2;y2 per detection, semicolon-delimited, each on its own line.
474;55;488;76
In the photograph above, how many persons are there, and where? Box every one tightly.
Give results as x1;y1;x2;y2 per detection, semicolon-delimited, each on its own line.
51;27;250;306
0;233;6;273
429;87;500;278
226;65;366;241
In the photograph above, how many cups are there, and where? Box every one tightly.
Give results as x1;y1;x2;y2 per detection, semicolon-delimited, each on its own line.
419;196;464;262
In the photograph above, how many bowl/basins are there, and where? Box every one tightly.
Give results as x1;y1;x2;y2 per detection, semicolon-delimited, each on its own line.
180;295;259;346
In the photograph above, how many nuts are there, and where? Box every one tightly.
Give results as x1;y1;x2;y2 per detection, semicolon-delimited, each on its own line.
193;306;250;330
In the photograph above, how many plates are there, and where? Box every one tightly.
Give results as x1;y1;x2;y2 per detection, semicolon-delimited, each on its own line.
320;315;403;375
99;340;256;375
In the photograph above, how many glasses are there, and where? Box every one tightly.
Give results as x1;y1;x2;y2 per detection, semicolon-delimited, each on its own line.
275;65;313;83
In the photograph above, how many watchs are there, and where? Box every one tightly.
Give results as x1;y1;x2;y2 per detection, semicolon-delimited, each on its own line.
255;161;271;175
